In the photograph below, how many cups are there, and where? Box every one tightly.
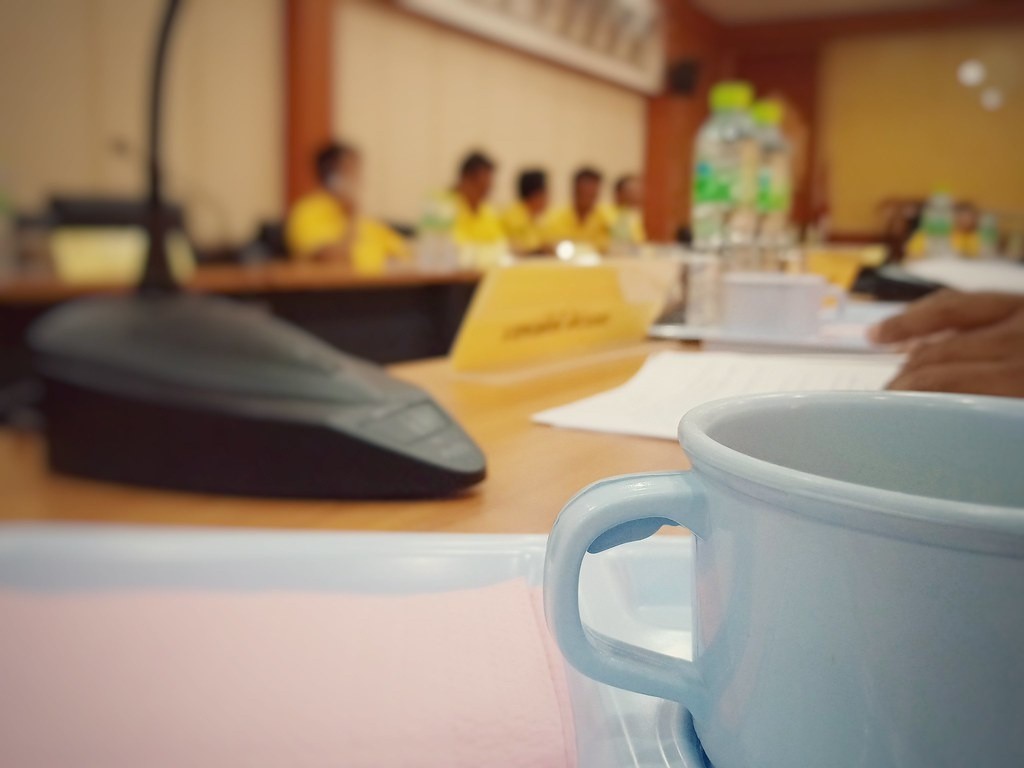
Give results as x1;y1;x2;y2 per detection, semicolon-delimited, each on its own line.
542;390;1024;768
726;271;848;336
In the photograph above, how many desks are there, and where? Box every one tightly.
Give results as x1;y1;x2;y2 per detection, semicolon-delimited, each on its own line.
0;320;900;768
0;261;478;423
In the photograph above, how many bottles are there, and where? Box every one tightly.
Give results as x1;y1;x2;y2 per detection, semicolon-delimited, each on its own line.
921;193;953;257
685;80;758;326
755;99;798;277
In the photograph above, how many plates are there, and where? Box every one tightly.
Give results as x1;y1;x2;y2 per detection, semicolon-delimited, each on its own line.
646;323;883;352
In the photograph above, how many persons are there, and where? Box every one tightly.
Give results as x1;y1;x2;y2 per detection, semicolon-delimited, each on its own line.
421;148;512;255
865;289;1024;400
285;144;411;268
500;169;647;255
